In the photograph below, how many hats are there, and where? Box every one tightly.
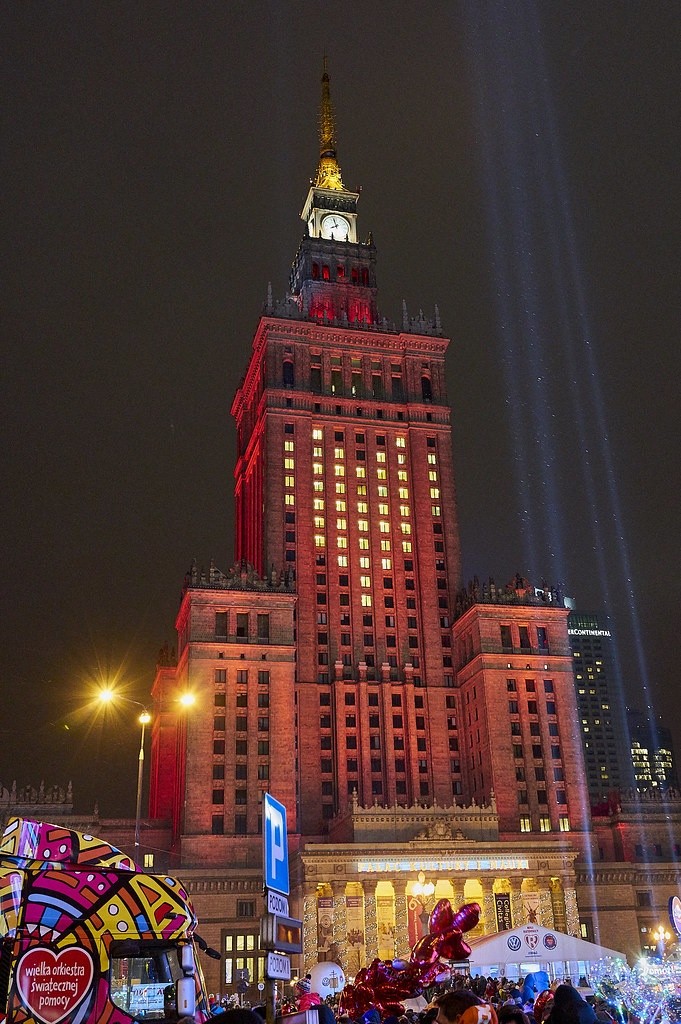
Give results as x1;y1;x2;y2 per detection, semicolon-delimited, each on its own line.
295;974;312;994
554;985;597;1024
362;1010;380;1024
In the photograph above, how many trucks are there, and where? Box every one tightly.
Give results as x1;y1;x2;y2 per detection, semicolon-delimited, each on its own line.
0;818;222;1024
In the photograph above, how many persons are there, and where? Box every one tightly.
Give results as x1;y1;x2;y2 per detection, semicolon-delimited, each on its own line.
208;974;681;1024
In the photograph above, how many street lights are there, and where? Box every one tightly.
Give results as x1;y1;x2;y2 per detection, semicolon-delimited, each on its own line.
100;687;197;867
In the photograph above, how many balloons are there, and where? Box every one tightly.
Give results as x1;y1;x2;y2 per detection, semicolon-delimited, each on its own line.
534;989;554;1024
339;898;482;1021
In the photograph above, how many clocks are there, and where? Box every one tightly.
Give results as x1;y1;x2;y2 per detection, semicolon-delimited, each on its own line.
320;214;350;240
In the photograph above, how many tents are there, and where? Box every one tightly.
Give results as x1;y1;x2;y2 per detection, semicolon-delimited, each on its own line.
399;922;628;987
